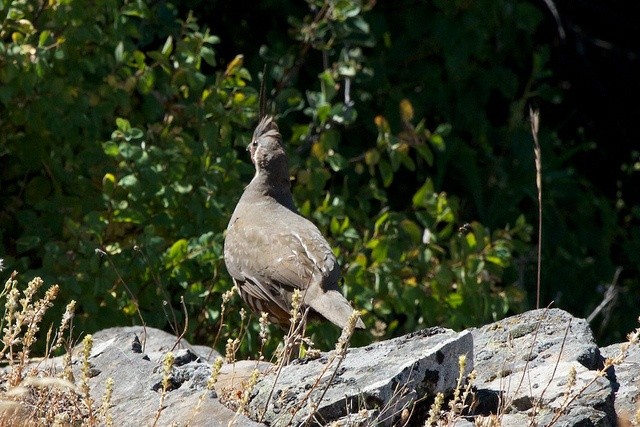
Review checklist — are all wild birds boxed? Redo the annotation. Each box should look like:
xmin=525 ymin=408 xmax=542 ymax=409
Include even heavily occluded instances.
xmin=222 ymin=113 xmax=367 ymax=366
